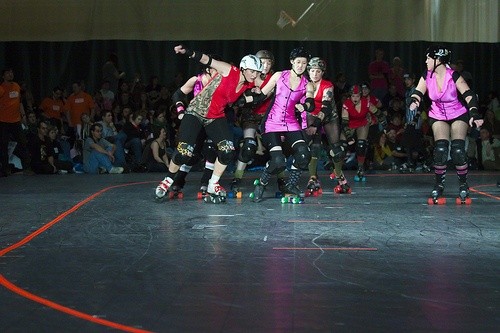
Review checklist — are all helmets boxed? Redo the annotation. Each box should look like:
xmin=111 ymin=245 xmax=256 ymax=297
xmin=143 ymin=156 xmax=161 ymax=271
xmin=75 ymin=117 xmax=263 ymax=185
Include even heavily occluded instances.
xmin=289 ymin=47 xmax=313 ymax=62
xmin=239 ymin=54 xmax=264 ymax=72
xmin=307 ymin=57 xmax=327 ymax=70
xmin=425 ymin=45 xmax=451 ymax=64
xmin=403 ymin=72 xmax=416 ymax=79
xmin=255 ymin=49 xmax=274 ymax=62
xmin=359 ymin=81 xmax=370 ymax=89
xmin=349 ymin=85 xmax=363 ymax=96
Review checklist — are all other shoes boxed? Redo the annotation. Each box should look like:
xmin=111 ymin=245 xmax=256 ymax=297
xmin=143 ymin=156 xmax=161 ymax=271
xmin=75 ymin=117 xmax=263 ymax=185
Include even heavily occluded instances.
xmin=385 ymin=165 xmax=430 ymax=172
xmin=0 ymin=164 xmax=155 ymax=176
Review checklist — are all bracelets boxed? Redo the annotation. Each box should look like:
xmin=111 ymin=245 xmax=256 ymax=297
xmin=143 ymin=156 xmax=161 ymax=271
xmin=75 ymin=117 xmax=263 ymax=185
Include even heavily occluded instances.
xmin=22 ymin=113 xmax=26 ymax=118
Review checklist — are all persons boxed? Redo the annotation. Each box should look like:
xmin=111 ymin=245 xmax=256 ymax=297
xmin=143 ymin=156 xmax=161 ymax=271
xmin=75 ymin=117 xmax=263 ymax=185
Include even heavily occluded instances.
xmin=409 ymin=47 xmax=484 ymax=198
xmin=101 ymin=52 xmax=127 ymax=105
xmin=226 ymin=48 xmax=500 ymax=174
xmin=156 ymin=44 xmax=262 ymax=198
xmin=304 ymin=56 xmax=348 ymax=191
xmin=329 ymin=84 xmax=388 ymax=181
xmin=230 ymin=50 xmax=305 ymax=197
xmin=172 ymin=54 xmax=228 ymax=195
xmin=7 ymin=72 xmax=208 ymax=176
xmin=232 ymin=47 xmax=315 ymax=200
xmin=0 ymin=65 xmax=35 ymax=177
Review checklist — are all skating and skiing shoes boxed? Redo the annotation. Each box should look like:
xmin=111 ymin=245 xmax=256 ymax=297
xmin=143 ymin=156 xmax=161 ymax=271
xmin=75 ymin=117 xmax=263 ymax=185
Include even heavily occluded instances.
xmin=305 ymin=176 xmax=322 ymax=197
xmin=334 ymin=176 xmax=351 ymax=194
xmin=153 ymin=177 xmax=174 ymax=202
xmin=330 ymin=169 xmax=337 ymax=180
xmin=428 ymin=186 xmax=446 ymax=205
xmin=197 ymin=179 xmax=209 ymax=199
xmin=169 ymin=178 xmax=185 ymax=199
xmin=455 ymin=183 xmax=472 ymax=205
xmin=354 ymin=168 xmax=366 ymax=182
xmin=204 ymin=180 xmax=226 ymax=204
xmin=228 ymin=179 xmax=242 ymax=199
xmin=280 ymin=183 xmax=304 ymax=204
xmin=248 ymin=178 xmax=268 ymax=202
